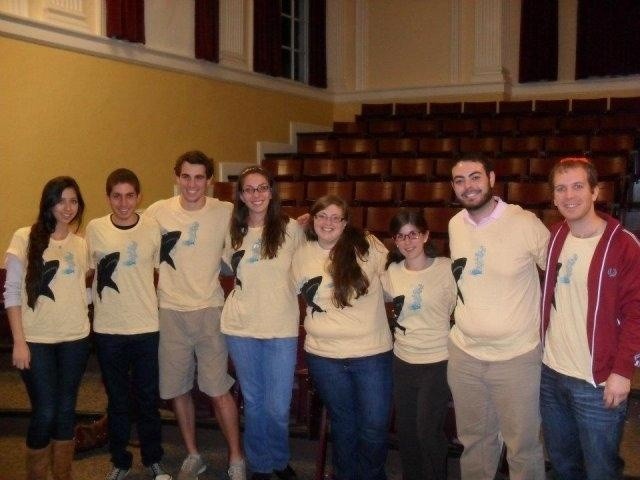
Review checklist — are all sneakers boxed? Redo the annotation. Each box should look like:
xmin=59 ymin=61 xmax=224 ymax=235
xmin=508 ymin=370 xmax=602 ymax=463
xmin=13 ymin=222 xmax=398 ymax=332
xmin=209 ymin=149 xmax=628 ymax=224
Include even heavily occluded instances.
xmin=227 ymin=459 xmax=247 ymax=480
xmin=250 ymin=471 xmax=271 ymax=479
xmin=104 ymin=466 xmax=132 ymax=480
xmin=149 ymin=461 xmax=173 ymax=480
xmin=176 ymin=455 xmax=208 ymax=480
xmin=272 ymin=464 xmax=297 ymax=480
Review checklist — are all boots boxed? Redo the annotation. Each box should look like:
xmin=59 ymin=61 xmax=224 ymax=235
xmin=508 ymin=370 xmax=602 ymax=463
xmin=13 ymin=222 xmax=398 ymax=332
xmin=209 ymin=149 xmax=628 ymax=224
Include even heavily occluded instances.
xmin=26 ymin=441 xmax=51 ymax=479
xmin=52 ymin=439 xmax=75 ymax=479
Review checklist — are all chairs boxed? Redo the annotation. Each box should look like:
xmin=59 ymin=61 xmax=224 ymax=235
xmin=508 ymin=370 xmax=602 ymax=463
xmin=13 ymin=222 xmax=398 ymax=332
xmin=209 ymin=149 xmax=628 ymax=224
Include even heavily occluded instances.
xmin=211 ymin=96 xmax=640 ymax=259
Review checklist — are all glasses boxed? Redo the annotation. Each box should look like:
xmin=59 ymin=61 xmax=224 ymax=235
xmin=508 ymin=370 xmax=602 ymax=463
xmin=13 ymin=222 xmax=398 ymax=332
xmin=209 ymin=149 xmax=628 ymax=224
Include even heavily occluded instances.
xmin=313 ymin=210 xmax=346 ymax=222
xmin=394 ymin=229 xmax=422 ymax=241
xmin=241 ymin=183 xmax=271 ymax=193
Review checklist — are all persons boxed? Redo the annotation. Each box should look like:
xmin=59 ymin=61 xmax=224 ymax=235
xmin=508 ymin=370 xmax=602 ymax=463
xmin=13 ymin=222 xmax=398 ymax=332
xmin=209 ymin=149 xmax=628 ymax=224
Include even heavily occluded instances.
xmin=141 ymin=150 xmax=313 ymax=480
xmin=540 ymin=158 xmax=640 ymax=480
xmin=84 ymin=168 xmax=169 ymax=480
xmin=379 ymin=210 xmax=456 ymax=480
xmin=3 ymin=175 xmax=90 ymax=480
xmin=445 ymin=151 xmax=549 ymax=480
xmin=218 ymin=166 xmax=309 ymax=480
xmin=288 ymin=195 xmax=395 ymax=480
